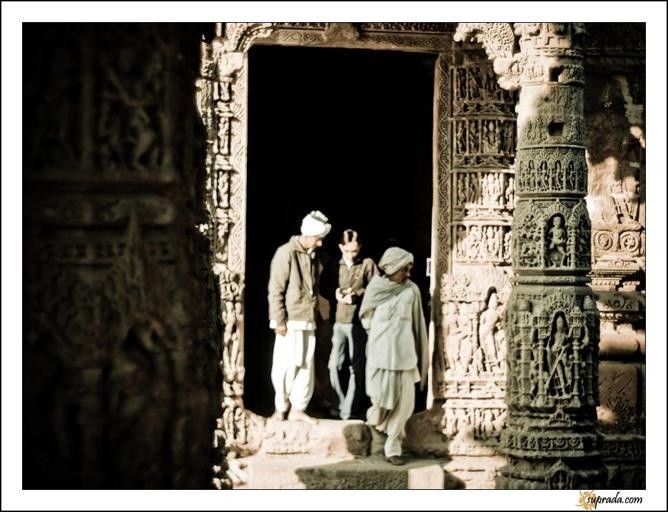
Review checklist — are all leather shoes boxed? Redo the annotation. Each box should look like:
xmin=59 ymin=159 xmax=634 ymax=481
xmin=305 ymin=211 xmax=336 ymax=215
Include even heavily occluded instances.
xmin=385 ymin=455 xmax=405 ymax=466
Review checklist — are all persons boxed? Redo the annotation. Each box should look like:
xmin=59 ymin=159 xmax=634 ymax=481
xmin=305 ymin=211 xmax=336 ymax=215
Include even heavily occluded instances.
xmin=546 ymin=316 xmax=575 ymax=397
xmin=357 ymin=244 xmax=429 ymax=467
xmin=437 ymin=52 xmax=515 ymax=443
xmin=547 ymin=213 xmax=567 ymax=268
xmin=314 ymin=223 xmax=380 ymax=418
xmin=266 ymin=210 xmax=333 ymax=426
